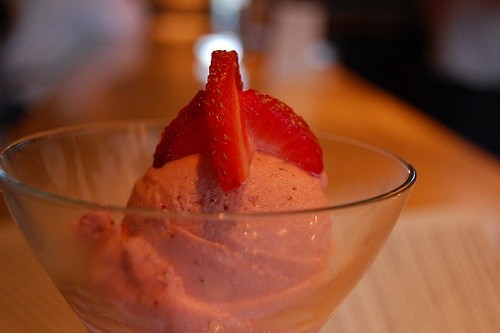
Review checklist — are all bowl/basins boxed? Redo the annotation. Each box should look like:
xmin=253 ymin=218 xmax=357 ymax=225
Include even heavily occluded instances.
xmin=0 ymin=119 xmax=416 ymax=332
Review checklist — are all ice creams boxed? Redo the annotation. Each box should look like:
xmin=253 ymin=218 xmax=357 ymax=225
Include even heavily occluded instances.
xmin=72 ymin=50 xmax=332 ymax=333
